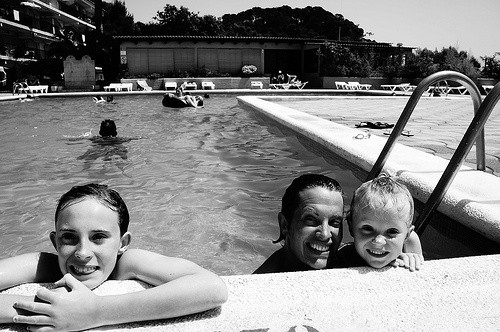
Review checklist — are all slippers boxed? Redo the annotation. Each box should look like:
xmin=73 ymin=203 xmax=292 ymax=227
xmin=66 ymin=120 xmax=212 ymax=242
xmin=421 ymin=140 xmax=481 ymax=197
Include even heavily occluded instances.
xmin=400 ymin=130 xmax=414 ymax=136
xmin=385 ymin=131 xmax=391 ymax=135
xmin=356 ymin=120 xmax=394 ymax=128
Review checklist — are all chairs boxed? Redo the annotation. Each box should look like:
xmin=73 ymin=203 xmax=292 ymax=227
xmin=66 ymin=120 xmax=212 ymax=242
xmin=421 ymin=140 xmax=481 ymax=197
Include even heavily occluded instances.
xmin=202 ymin=81 xmax=215 ymax=89
xmin=184 ymin=82 xmax=197 ymax=89
xmin=165 ymin=82 xmax=178 ymax=90
xmin=251 ymin=81 xmax=263 ymax=88
xmin=380 ymin=83 xmax=417 ymax=92
xmin=482 ymin=84 xmax=494 ymax=94
xmin=424 ymin=85 xmax=468 ymax=94
xmin=18 ymin=85 xmax=49 ymax=94
xmin=335 ymin=81 xmax=372 ymax=90
xmin=269 ymin=75 xmax=309 ymax=89
xmin=136 ymin=80 xmax=152 ymax=91
xmin=104 ymin=82 xmax=134 ymax=92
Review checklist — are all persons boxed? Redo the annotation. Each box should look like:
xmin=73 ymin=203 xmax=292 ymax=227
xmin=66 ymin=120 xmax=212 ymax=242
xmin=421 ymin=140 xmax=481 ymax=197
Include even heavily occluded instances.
xmin=93 ymin=95 xmax=114 ymax=104
xmin=169 ymin=86 xmax=203 ymax=108
xmin=0 ymin=183 xmax=228 ymax=332
xmin=99 ymin=119 xmax=118 ymax=137
xmin=251 ymin=172 xmax=424 ymax=275
